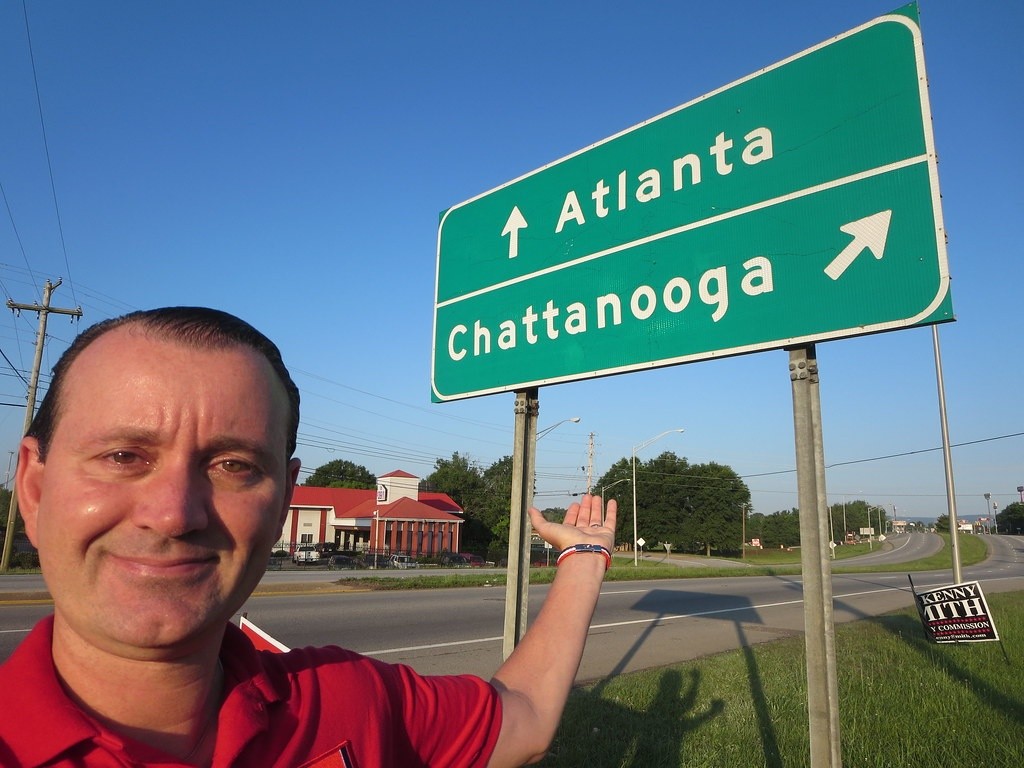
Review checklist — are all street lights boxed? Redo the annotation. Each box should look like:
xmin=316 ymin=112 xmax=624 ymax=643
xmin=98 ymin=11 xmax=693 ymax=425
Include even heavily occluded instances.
xmin=631 ymin=428 xmax=685 ymax=566
xmin=601 ymin=478 xmax=631 ymax=525
xmin=843 ymin=490 xmax=863 ymax=544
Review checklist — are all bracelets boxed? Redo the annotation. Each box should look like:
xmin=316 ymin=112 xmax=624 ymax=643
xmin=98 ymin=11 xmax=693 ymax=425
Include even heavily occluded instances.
xmin=556 ymin=543 xmax=611 ymax=570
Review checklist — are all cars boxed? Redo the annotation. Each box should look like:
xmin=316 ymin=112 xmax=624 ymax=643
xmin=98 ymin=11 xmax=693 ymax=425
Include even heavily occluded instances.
xmin=327 ymin=556 xmax=364 ymax=570
xmin=315 ymin=541 xmax=338 ymax=557
xmin=470 ymin=556 xmax=487 ymax=567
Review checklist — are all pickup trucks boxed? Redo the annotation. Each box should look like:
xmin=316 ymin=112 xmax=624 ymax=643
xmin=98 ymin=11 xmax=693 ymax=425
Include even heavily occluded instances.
xmin=390 ymin=554 xmax=417 ymax=569
xmin=292 ymin=545 xmax=321 ymax=566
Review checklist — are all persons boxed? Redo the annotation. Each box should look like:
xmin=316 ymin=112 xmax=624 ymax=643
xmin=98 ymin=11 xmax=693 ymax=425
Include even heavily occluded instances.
xmin=0 ymin=307 xmax=617 ymax=768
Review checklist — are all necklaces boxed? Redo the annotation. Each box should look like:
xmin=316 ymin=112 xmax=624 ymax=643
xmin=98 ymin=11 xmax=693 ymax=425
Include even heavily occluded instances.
xmin=182 ymin=657 xmax=224 ymax=761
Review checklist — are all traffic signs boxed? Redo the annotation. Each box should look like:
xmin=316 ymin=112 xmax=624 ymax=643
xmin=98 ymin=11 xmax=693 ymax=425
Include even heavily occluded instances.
xmin=429 ymin=1 xmax=958 ymax=408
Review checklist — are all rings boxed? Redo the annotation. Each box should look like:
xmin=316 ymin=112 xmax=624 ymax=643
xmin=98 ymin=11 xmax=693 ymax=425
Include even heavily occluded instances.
xmin=590 ymin=524 xmax=598 ymax=527
